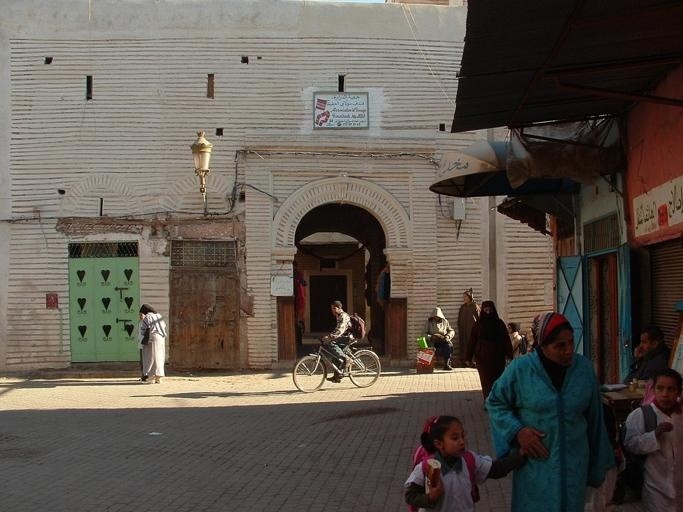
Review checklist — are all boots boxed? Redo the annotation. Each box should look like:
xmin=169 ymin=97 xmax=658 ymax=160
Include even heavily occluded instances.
xmin=443 ymin=359 xmax=452 ymax=370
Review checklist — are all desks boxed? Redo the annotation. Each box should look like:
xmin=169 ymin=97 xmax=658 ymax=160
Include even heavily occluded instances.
xmin=600 ymin=383 xmax=648 ymax=505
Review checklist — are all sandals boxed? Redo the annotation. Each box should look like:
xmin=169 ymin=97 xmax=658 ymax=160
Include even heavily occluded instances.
xmin=154 ymin=378 xmax=160 ymax=383
xmin=144 ymin=379 xmax=155 ymax=384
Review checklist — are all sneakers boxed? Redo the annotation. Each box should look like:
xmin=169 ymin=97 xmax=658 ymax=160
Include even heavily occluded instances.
xmin=140 ymin=376 xmax=148 ymax=381
xmin=342 ymin=358 xmax=353 ymax=368
xmin=327 ymin=376 xmax=341 ymax=383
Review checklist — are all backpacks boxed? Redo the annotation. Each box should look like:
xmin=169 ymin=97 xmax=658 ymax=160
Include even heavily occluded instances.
xmin=346 ymin=313 xmax=366 ymax=339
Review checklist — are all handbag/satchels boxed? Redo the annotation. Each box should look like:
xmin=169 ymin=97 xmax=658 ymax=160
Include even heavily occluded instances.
xmin=141 ymin=328 xmax=150 ymax=345
xmin=610 ymin=453 xmax=642 ymax=504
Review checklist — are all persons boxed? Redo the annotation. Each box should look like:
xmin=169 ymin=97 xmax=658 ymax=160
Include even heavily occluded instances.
xmin=506 ymin=320 xmax=528 ymax=360
xmin=621 ymin=326 xmax=671 ymax=384
xmin=619 ymin=367 xmax=682 ymax=512
xmin=464 ymin=299 xmax=514 ymax=412
xmin=294 ymin=317 xmax=305 ymax=358
xmin=137 ymin=311 xmax=148 ymax=382
xmin=137 ymin=303 xmax=168 ymax=385
xmin=320 ymin=300 xmax=357 ymax=383
xmin=422 ymin=306 xmax=455 ymax=370
xmin=456 ymin=287 xmax=481 ymax=367
xmin=401 ymin=413 xmax=529 ymax=511
xmin=484 ymin=311 xmax=616 ymax=512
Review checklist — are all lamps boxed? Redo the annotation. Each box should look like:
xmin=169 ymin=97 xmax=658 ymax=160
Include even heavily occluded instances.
xmin=187 ymin=129 xmax=215 ymax=213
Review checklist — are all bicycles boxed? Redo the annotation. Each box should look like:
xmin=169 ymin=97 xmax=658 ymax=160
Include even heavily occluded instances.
xmin=291 ymin=334 xmax=383 ymax=393
